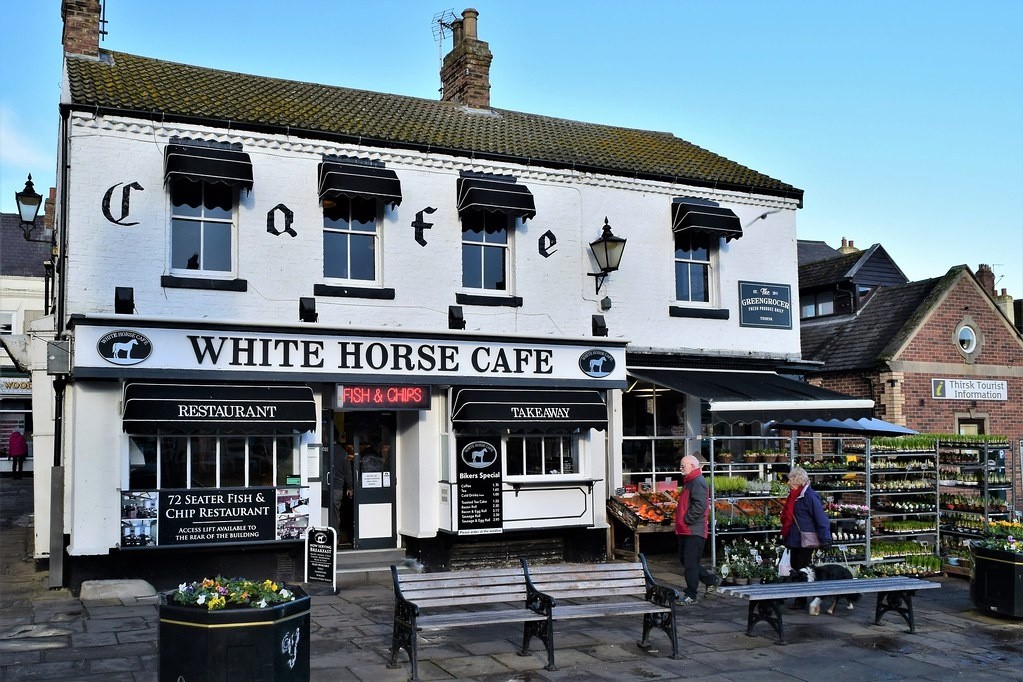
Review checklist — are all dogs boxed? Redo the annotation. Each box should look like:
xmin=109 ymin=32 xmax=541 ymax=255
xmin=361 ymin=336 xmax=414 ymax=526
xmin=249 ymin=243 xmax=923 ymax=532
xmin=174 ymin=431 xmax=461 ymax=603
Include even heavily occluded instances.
xmin=788 ymin=564 xmax=862 ymax=615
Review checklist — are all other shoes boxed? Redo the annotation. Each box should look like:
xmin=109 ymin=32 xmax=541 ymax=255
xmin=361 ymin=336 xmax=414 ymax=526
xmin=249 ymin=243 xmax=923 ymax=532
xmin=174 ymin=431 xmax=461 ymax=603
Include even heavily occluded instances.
xmin=13 ymin=475 xmax=22 ymax=480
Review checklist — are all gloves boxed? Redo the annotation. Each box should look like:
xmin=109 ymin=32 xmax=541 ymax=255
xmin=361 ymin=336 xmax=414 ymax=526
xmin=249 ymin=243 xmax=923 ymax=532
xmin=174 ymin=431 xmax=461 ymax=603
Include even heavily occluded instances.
xmin=820 ymin=542 xmax=832 ymax=552
xmin=8 ymin=455 xmax=11 ymax=460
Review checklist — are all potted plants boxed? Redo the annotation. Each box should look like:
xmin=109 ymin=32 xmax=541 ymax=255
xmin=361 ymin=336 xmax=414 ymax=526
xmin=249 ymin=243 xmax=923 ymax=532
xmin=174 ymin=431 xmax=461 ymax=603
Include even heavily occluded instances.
xmin=743 ymin=449 xmax=761 ymax=462
xmin=777 ymin=449 xmax=790 ymax=462
xmin=759 ymin=449 xmax=778 ymax=462
xmin=719 ymin=447 xmax=733 ymax=462
xmin=940 ymin=433 xmax=1010 ymax=568
xmin=705 ymin=472 xmax=790 ymax=529
xmin=795 ymin=436 xmax=937 ymax=576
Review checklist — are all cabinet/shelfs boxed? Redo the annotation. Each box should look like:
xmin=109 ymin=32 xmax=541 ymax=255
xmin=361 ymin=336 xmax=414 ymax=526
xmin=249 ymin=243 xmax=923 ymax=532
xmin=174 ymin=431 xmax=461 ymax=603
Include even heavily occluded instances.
xmin=791 ymin=435 xmax=869 ymax=567
xmin=867 ymin=440 xmax=939 ymax=575
xmin=684 ymin=435 xmax=791 ymax=586
xmin=940 ymin=435 xmax=1016 ymax=577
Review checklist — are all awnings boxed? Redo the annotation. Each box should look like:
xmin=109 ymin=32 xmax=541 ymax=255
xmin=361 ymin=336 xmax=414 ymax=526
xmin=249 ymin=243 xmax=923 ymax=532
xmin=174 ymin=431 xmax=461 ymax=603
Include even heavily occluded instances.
xmin=164 ymin=144 xmax=253 ymax=198
xmin=451 ymin=386 xmax=608 ymax=433
xmin=122 ymin=379 xmax=317 ymax=434
xmin=457 ymin=177 xmax=537 ymax=224
xmin=318 ymin=162 xmax=402 ymax=211
xmin=627 ymin=366 xmax=875 ymax=411
xmin=672 ymin=203 xmax=743 ymax=243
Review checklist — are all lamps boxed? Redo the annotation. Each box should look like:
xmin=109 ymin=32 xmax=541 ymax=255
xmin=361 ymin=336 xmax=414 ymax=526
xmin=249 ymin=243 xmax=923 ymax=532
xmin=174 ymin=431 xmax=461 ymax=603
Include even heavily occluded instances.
xmin=587 ymin=217 xmax=627 ymax=295
xmin=15 ymin=172 xmax=57 ymax=246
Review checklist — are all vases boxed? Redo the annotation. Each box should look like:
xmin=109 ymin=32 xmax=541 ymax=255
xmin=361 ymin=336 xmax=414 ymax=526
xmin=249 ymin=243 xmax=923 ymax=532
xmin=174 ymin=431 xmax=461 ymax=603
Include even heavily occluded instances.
xmin=726 ymin=577 xmax=733 ymax=582
xmin=750 ymin=578 xmax=763 ymax=583
xmin=735 ymin=578 xmax=748 ymax=584
xmin=156 ymin=580 xmax=313 ymax=682
xmin=967 ymin=539 xmax=1023 ymax=624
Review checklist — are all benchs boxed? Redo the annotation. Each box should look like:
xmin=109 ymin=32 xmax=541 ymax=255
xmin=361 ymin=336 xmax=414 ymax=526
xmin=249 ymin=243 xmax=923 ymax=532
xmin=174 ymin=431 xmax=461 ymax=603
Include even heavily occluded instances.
xmin=713 ymin=575 xmax=946 ymax=646
xmin=389 ymin=552 xmax=685 ymax=682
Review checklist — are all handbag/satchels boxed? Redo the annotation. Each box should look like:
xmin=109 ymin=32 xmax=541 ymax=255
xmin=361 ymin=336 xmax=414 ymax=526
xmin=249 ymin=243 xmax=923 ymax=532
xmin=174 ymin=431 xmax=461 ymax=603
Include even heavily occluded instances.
xmin=778 ymin=548 xmax=791 ymax=577
xmin=800 ymin=532 xmax=820 ymax=549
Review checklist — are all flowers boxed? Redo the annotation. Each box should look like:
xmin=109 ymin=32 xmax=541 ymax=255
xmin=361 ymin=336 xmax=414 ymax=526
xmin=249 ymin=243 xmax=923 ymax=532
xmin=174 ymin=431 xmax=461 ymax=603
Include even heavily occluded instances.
xmin=170 ymin=569 xmax=296 ymax=608
xmin=980 ymin=519 xmax=1023 ymax=553
xmin=719 ymin=535 xmax=783 ymax=577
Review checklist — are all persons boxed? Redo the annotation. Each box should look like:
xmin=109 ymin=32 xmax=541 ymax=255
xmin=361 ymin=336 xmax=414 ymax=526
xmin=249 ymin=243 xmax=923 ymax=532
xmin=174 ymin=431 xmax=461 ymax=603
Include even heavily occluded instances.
xmin=673 ymin=455 xmax=720 ymax=606
xmin=121 ymin=503 xmax=158 ymax=519
xmin=123 ymin=529 xmax=149 ymax=547
xmin=781 ymin=467 xmax=833 ymax=610
xmin=8 ymin=427 xmax=27 ymax=479
xmin=323 ymin=423 xmax=354 ymax=547
xmin=350 ymin=438 xmax=384 ymax=474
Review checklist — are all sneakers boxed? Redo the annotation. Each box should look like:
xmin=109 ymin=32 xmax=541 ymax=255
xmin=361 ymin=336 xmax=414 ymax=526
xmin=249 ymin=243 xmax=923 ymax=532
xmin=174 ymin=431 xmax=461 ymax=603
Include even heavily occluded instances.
xmin=676 ymin=596 xmax=698 ymax=606
xmin=704 ymin=581 xmax=719 ymax=598
